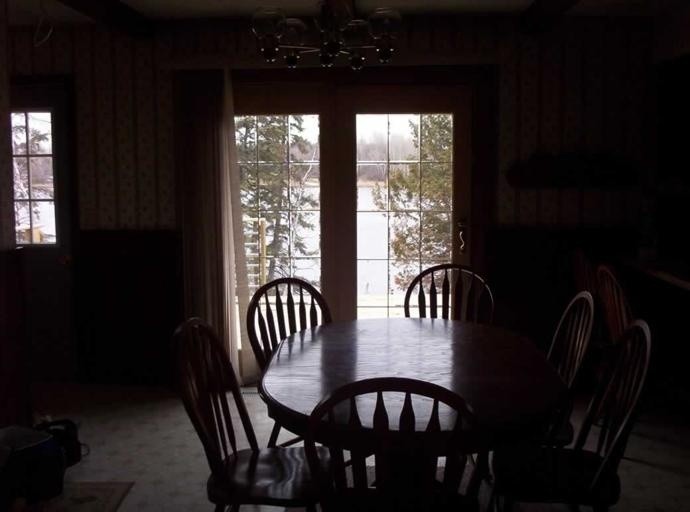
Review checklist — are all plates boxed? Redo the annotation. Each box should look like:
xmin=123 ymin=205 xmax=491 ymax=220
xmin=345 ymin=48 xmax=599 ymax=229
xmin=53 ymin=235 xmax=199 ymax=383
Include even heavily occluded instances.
xmin=0 ymin=424 xmax=53 ymax=452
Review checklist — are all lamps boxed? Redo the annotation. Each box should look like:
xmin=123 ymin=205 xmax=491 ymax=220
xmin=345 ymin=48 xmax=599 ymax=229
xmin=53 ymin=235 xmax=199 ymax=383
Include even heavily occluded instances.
xmin=251 ymin=0 xmax=403 ymax=70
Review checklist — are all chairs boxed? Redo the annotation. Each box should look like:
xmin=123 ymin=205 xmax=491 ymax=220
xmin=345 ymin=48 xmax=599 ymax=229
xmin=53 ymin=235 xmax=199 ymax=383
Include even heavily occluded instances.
xmin=171 ymin=264 xmax=653 ymax=512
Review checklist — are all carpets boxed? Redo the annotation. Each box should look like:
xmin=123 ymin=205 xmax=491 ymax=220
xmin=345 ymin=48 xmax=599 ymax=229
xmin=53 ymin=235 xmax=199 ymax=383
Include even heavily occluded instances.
xmin=31 ymin=481 xmax=135 ymax=512
